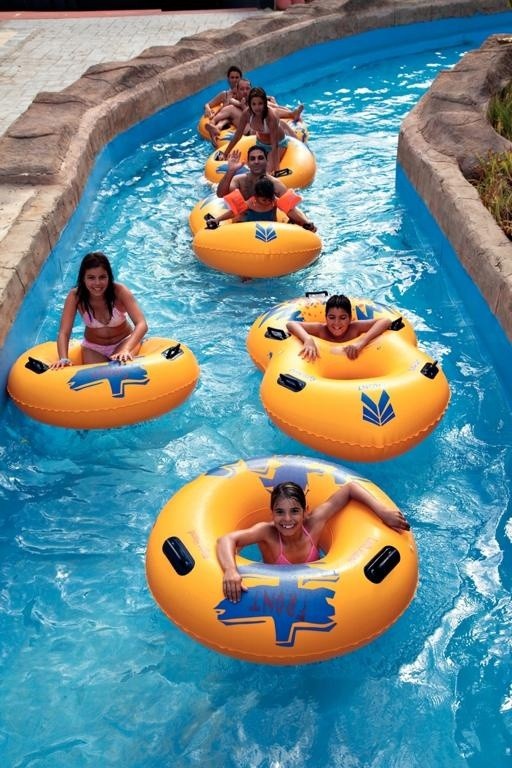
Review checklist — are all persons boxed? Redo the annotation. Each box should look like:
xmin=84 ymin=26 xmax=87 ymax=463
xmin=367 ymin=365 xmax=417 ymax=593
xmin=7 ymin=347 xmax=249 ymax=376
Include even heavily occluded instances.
xmin=215 ymin=479 xmax=411 ymax=604
xmin=48 ymin=252 xmax=147 ymax=371
xmin=203 ymin=66 xmax=318 ymax=286
xmin=286 ymin=295 xmax=393 ymax=363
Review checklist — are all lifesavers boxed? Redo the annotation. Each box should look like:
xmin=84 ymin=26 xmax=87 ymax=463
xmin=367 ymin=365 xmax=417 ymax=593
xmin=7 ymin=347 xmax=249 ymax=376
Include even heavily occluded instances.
xmin=249 ymin=292 xmax=451 ymax=464
xmin=146 ymin=454 xmax=417 ymax=664
xmin=8 ymin=337 xmax=198 ymax=428
xmin=188 ymin=98 xmax=320 ymax=278
